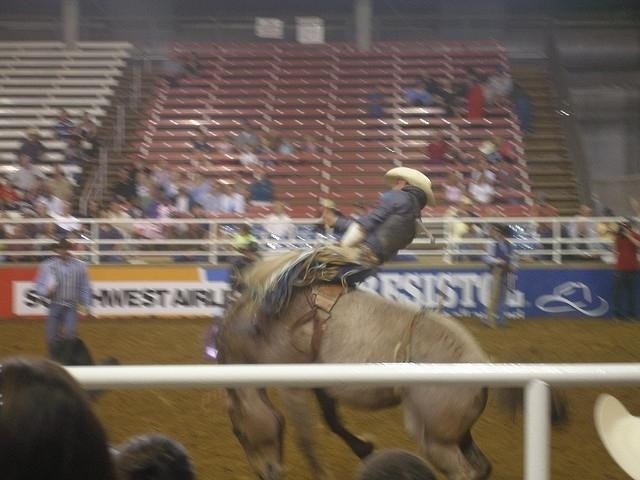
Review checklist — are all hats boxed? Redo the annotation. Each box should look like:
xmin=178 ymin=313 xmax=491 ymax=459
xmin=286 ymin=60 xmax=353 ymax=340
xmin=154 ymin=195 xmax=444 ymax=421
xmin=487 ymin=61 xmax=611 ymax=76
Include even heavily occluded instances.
xmin=594 ymin=392 xmax=639 ymax=479
xmin=384 ymin=167 xmax=437 ymax=207
xmin=52 ymin=238 xmax=73 ymax=249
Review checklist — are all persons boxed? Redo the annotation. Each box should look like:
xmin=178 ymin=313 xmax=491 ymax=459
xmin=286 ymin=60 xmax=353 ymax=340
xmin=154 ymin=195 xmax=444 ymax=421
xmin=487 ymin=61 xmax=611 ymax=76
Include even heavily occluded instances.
xmin=253 ymin=165 xmax=436 ymax=327
xmin=352 ymin=449 xmax=437 ymax=478
xmin=538 ymin=211 xmax=639 ymax=265
xmin=609 ymin=217 xmax=640 ymax=319
xmin=314 ymin=200 xmax=341 ymax=236
xmin=484 ymin=224 xmax=519 ymax=326
xmin=0 ymin=110 xmax=99 ymax=263
xmin=114 ymin=433 xmax=197 ymax=480
xmin=404 ymin=61 xmax=518 ymax=207
xmin=33 ymin=238 xmax=98 ymax=346
xmin=1 ymin=356 xmax=117 ymax=479
xmin=95 ymin=121 xmax=274 ymax=264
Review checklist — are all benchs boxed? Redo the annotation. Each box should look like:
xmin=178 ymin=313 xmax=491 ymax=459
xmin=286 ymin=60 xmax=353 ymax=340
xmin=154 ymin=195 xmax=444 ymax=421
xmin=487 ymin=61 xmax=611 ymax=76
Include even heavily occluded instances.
xmin=128 ymin=39 xmax=540 ymax=235
xmin=0 ymin=36 xmax=139 ymax=232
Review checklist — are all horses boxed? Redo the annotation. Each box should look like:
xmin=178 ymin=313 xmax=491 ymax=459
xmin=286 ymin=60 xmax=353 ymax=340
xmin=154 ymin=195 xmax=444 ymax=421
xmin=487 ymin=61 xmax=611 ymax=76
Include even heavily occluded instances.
xmin=217 ymin=250 xmax=572 ymax=479
